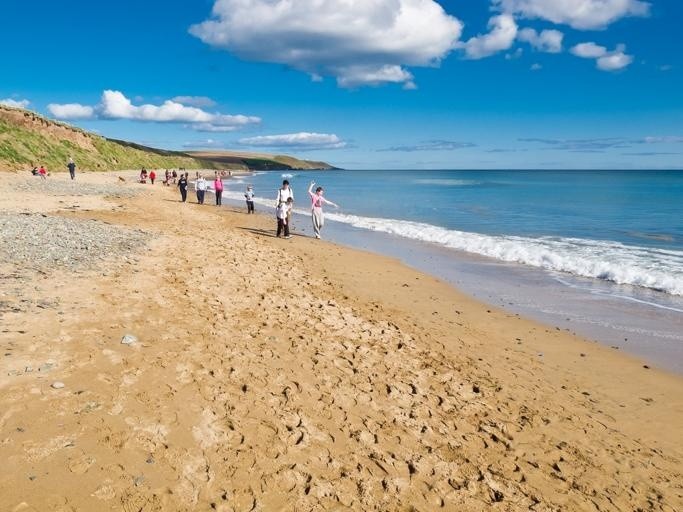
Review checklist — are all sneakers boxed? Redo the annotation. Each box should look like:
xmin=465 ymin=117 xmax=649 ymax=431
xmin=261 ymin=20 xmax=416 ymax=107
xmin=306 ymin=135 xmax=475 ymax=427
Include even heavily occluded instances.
xmin=277 ymin=233 xmax=292 ymax=239
xmin=316 ymin=235 xmax=321 ymax=239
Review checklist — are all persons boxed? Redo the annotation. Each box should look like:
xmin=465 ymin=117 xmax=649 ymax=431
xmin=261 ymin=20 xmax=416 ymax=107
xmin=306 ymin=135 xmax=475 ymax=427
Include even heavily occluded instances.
xmin=213 ymin=169 xmax=233 ymax=177
xmin=184 ymin=171 xmax=189 ymax=180
xmin=66 ymin=158 xmax=76 ymax=179
xmin=195 ymin=174 xmax=207 ymax=205
xmin=31 ymin=166 xmax=47 ymax=182
xmin=244 ymin=185 xmax=254 ymax=214
xmin=214 ymin=175 xmax=223 ymax=207
xmin=163 ymin=168 xmax=177 ymax=187
xmin=140 ymin=167 xmax=155 ymax=185
xmin=274 ymin=179 xmax=294 ymax=239
xmin=308 ymin=179 xmax=339 ymax=239
xmin=178 ymin=175 xmax=188 ymax=203
xmin=195 ymin=170 xmax=200 ymax=178
